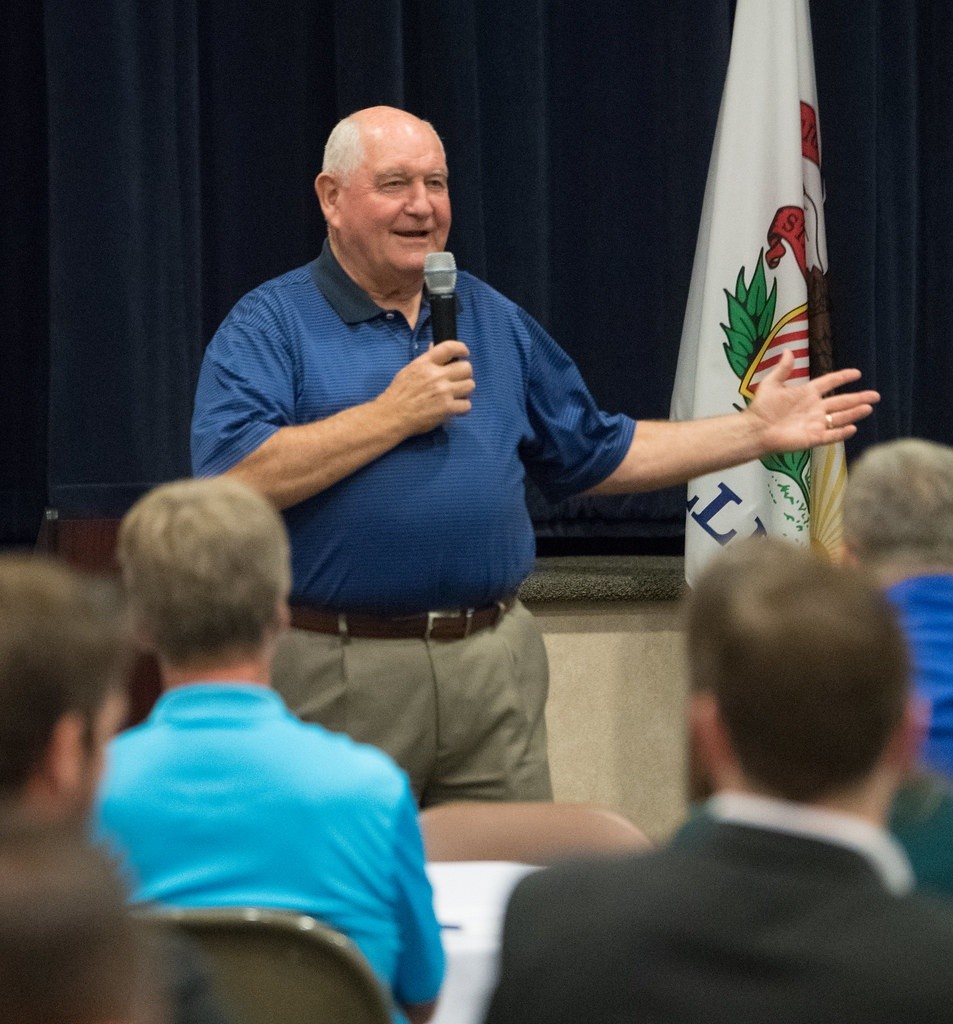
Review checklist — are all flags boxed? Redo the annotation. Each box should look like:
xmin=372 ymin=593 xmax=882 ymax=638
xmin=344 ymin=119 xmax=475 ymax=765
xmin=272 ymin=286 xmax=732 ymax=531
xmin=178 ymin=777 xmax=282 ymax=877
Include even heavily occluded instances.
xmin=668 ymin=1 xmax=829 ymax=588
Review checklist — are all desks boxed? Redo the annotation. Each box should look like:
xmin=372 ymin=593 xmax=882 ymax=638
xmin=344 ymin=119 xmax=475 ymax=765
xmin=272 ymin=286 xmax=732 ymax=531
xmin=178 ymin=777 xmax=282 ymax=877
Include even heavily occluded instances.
xmin=51 ymin=511 xmax=694 ymax=606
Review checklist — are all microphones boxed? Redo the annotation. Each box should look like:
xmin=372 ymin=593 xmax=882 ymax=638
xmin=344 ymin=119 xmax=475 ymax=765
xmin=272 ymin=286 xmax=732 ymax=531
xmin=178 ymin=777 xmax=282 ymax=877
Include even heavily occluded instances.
xmin=422 ymin=252 xmax=468 ymax=416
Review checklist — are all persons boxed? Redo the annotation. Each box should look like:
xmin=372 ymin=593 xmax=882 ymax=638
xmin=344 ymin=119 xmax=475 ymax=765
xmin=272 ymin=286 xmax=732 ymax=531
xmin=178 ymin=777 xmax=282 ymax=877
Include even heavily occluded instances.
xmin=189 ymin=103 xmax=882 ymax=811
xmin=92 ymin=472 xmax=448 ymax=1022
xmin=3 ymin=826 xmax=158 ymax=1020
xmin=840 ymin=437 xmax=952 ymax=781
xmin=3 ymin=554 xmax=134 ymax=834
xmin=479 ymin=532 xmax=952 ymax=1020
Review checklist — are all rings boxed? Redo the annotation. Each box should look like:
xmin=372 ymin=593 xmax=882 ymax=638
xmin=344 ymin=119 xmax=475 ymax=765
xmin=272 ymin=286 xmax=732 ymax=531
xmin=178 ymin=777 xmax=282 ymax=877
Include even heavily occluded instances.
xmin=825 ymin=415 xmax=833 ymax=429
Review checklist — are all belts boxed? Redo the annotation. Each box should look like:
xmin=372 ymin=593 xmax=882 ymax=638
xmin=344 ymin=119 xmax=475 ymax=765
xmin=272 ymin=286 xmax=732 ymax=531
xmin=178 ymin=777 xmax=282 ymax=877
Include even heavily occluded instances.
xmin=287 ymin=591 xmax=527 ymax=642
